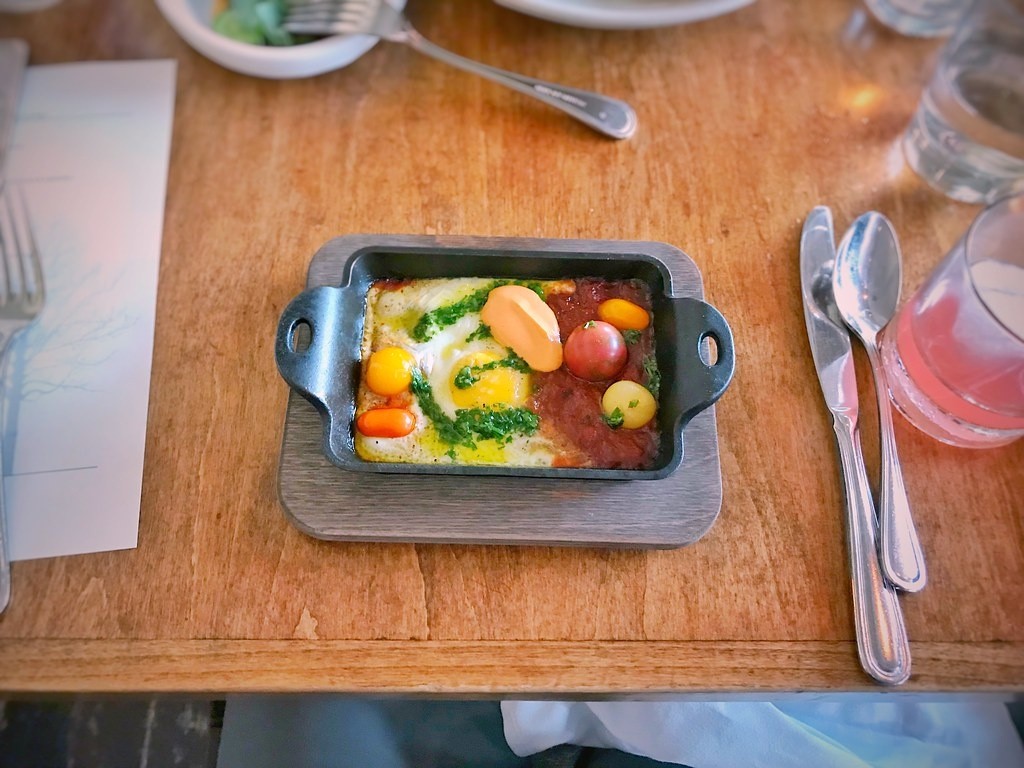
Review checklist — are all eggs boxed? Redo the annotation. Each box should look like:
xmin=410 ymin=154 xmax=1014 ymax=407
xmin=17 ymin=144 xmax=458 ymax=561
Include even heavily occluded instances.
xmin=356 ymin=277 xmax=564 ymax=467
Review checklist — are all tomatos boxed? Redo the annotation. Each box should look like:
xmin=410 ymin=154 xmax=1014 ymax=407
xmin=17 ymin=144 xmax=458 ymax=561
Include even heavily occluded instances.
xmin=356 ymin=299 xmax=655 ymax=438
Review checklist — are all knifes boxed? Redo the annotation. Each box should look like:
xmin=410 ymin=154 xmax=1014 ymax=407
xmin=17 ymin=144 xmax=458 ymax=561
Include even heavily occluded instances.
xmin=799 ymin=206 xmax=913 ymax=686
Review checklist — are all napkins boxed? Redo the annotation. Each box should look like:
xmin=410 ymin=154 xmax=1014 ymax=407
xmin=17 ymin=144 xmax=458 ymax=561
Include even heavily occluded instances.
xmin=0 ymin=59 xmax=175 ymax=563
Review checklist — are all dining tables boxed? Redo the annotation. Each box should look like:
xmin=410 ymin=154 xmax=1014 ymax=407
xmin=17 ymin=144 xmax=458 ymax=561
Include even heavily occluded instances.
xmin=0 ymin=0 xmax=1024 ymax=697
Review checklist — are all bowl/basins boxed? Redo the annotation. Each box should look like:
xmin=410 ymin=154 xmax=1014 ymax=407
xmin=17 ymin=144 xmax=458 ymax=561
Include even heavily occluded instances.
xmin=155 ymin=0 xmax=405 ymax=79
xmin=496 ymin=0 xmax=756 ymax=28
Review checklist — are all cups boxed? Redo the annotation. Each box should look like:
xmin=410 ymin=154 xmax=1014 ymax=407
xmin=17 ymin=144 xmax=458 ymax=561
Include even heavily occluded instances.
xmin=900 ymin=0 xmax=1024 ymax=202
xmin=863 ymin=0 xmax=966 ymax=37
xmin=882 ymin=191 xmax=1024 ymax=450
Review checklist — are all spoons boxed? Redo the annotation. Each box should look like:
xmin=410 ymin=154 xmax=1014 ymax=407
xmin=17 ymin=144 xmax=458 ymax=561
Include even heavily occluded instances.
xmin=832 ymin=209 xmax=927 ymax=594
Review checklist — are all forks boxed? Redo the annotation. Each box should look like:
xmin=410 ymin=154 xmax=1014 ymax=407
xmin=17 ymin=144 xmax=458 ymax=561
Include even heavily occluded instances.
xmin=283 ymin=0 xmax=635 ymax=139
xmin=0 ymin=181 xmax=46 ymax=616
